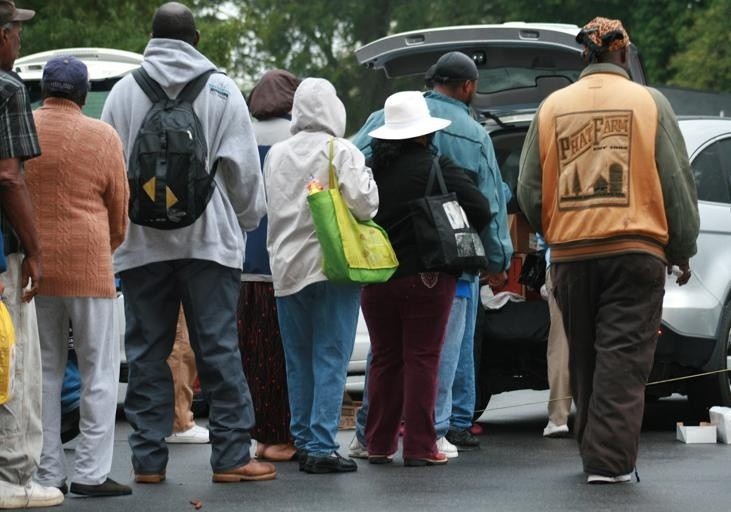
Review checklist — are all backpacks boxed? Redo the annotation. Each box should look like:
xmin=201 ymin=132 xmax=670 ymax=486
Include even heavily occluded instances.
xmin=127 ymin=67 xmax=219 ymax=230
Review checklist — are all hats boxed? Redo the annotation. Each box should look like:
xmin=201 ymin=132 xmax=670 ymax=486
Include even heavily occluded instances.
xmin=42 ymin=56 xmax=88 ymax=99
xmin=1 ymin=2 xmax=36 ymax=25
xmin=368 ymin=91 xmax=451 ymax=140
xmin=247 ymin=68 xmax=300 ymax=119
xmin=434 ymin=51 xmax=478 ymax=82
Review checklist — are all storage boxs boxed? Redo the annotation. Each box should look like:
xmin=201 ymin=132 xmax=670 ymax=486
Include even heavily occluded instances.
xmin=507 ymin=210 xmax=535 ymax=253
xmin=491 ymin=252 xmax=536 ymax=302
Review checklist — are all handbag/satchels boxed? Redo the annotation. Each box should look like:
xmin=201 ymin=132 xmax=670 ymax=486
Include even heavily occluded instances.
xmin=408 ymin=156 xmax=488 ymax=273
xmin=518 ymin=249 xmax=547 ymax=291
xmin=60 ymin=343 xmax=81 ymax=443
xmin=307 ymin=139 xmax=400 ymax=286
xmin=2 ymin=296 xmax=16 ymax=404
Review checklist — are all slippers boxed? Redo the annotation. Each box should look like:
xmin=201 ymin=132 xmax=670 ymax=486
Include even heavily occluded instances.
xmin=254 ymin=449 xmax=304 ymax=462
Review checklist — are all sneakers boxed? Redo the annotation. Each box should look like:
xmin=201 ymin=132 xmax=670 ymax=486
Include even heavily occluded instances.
xmin=446 ymin=429 xmax=480 ymax=450
xmin=0 ymin=482 xmax=65 ymax=508
xmin=164 ymin=426 xmax=210 ymax=443
xmin=435 ymin=437 xmax=458 ymax=458
xmin=348 ymin=435 xmax=369 ymax=458
xmin=587 ymin=472 xmax=631 ymax=483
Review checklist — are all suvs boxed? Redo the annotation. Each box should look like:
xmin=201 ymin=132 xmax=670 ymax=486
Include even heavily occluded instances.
xmin=353 ymin=19 xmax=731 ymax=434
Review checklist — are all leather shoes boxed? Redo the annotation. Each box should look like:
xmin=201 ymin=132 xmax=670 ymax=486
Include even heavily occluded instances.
xmin=403 ymin=450 xmax=447 ymax=466
xmin=213 ymin=451 xmax=277 ymax=482
xmin=368 ymin=454 xmax=393 ymax=464
xmin=135 ymin=470 xmax=166 ymax=483
xmin=542 ymin=420 xmax=569 ymax=436
xmin=302 ymin=450 xmax=357 ymax=474
xmin=71 ymin=478 xmax=131 ymax=497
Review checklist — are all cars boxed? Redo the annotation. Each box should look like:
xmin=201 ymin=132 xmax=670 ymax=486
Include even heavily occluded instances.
xmin=9 ymin=45 xmax=372 ymax=413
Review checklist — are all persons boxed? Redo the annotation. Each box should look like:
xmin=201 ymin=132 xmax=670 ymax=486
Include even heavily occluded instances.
xmin=542 ymin=248 xmax=574 ymax=439
xmin=422 ymin=62 xmax=512 ymax=449
xmin=100 ymin=0 xmax=276 ymax=483
xmin=236 ymin=67 xmax=301 ymax=464
xmin=0 ymin=0 xmax=64 ymax=509
xmin=515 ymin=14 xmax=701 ymax=485
xmin=359 ymin=89 xmax=492 ymax=466
xmin=18 ymin=53 xmax=132 ymax=498
xmin=262 ymin=76 xmax=380 ymax=475
xmin=348 ymin=51 xmax=514 ymax=461
